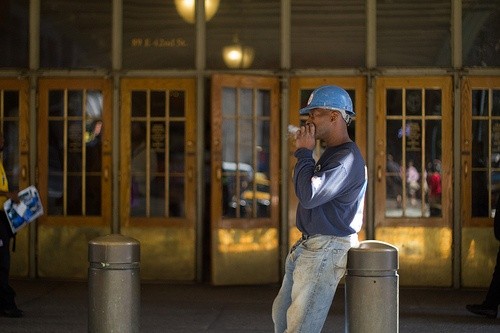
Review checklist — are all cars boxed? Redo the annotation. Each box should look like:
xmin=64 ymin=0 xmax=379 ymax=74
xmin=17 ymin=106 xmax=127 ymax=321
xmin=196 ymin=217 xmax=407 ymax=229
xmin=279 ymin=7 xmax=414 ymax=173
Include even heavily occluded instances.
xmin=77 ymin=128 xmax=254 ymax=212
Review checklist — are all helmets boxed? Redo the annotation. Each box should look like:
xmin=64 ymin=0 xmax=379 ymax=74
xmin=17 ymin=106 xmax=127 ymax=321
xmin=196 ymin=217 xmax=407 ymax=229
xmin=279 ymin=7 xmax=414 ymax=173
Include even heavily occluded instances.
xmin=299 ymin=85 xmax=355 ymax=119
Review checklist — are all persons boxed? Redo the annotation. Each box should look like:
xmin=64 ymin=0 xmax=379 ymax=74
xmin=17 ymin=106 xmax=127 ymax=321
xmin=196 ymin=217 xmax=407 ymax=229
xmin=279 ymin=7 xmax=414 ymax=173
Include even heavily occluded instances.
xmin=0 ymin=133 xmax=24 ymax=318
xmin=384 ymin=154 xmax=442 ymax=217
xmin=273 ymin=85 xmax=368 ymax=333
xmin=465 ymin=194 xmax=500 ymax=317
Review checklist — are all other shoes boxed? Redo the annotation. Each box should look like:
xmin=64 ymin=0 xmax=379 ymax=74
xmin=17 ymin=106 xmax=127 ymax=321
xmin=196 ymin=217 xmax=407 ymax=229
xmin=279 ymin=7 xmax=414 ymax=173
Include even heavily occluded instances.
xmin=0 ymin=306 xmax=24 ymax=317
xmin=465 ymin=303 xmax=498 ymax=319
